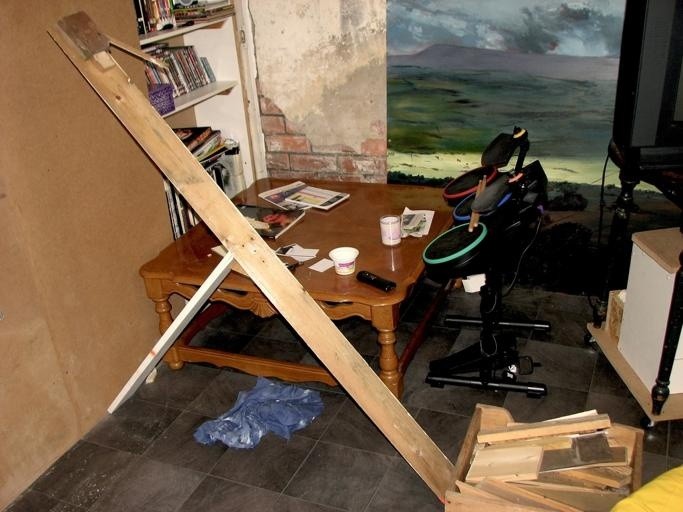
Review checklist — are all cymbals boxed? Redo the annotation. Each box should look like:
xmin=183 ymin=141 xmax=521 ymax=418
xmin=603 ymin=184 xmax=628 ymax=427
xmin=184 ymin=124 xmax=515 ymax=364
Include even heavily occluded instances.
xmin=471 ymin=175 xmax=509 ymax=213
xmin=481 ymin=133 xmax=518 ymax=167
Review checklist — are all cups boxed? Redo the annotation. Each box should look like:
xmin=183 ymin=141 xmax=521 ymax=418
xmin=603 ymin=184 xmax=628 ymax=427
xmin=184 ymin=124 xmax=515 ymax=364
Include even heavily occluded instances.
xmin=328 ymin=247 xmax=359 ymax=275
xmin=379 ymin=214 xmax=401 ymax=246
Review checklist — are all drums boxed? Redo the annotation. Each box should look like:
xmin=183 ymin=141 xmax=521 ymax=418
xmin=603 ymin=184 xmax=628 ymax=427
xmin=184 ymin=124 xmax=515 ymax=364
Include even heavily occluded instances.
xmin=423 ymin=223 xmax=488 ymax=264
xmin=453 ymin=189 xmax=512 ymax=221
xmin=443 ymin=166 xmax=497 ymax=198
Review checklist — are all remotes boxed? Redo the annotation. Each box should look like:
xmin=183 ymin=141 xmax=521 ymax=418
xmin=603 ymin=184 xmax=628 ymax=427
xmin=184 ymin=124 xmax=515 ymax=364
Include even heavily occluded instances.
xmin=357 ymin=271 xmax=396 ymax=293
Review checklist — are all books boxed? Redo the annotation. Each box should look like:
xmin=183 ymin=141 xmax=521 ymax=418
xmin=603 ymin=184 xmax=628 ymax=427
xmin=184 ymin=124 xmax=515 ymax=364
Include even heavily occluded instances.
xmin=234 ymin=203 xmax=306 ymax=242
xmin=132 ymin=1 xmax=248 ymax=243
xmin=287 ymin=186 xmax=350 ymax=212
xmin=257 ymin=179 xmax=316 ymax=212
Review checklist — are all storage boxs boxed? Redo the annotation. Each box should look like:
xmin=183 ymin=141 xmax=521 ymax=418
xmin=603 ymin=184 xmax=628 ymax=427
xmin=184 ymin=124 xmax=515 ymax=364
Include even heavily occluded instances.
xmin=618 ymin=225 xmax=681 ymax=396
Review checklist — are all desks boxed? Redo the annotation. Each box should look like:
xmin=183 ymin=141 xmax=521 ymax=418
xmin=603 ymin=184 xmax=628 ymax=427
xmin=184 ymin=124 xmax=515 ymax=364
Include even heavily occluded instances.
xmin=139 ymin=179 xmax=456 ymax=401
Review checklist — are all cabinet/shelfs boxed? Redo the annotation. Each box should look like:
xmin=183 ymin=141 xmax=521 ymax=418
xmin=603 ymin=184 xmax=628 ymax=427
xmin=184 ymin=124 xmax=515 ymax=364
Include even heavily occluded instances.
xmin=132 ymin=1 xmax=258 ymax=241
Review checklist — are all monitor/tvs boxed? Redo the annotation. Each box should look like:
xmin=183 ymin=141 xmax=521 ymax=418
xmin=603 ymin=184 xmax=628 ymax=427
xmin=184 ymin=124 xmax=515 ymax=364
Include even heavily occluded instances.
xmin=611 ymin=0 xmax=683 ymax=170
